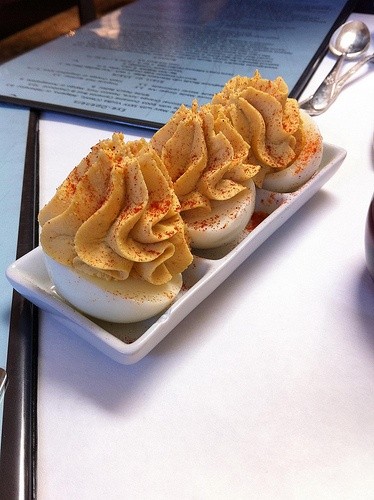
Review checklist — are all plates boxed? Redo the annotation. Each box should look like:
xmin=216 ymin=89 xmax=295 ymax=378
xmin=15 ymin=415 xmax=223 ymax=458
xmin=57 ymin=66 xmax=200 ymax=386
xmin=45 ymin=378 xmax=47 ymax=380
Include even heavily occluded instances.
xmin=5 ymin=137 xmax=349 ymax=368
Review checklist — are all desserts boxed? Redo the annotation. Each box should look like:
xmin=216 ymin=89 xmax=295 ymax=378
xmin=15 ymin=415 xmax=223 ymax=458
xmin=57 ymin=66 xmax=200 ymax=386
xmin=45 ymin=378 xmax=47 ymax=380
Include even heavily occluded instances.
xmin=35 ymin=67 xmax=324 ymax=325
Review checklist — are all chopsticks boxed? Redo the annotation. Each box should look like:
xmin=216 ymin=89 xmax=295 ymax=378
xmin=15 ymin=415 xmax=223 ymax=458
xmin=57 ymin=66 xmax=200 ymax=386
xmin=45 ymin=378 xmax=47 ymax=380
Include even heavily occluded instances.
xmin=0 ymin=106 xmax=43 ymax=500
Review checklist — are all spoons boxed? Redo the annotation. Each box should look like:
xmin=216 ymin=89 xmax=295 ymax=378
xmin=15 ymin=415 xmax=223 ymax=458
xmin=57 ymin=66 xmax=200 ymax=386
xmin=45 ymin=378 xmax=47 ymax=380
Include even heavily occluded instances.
xmin=312 ymin=18 xmax=371 ymax=109
